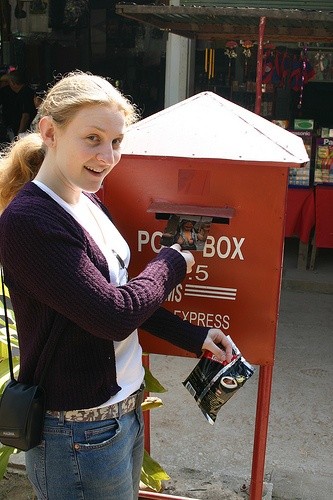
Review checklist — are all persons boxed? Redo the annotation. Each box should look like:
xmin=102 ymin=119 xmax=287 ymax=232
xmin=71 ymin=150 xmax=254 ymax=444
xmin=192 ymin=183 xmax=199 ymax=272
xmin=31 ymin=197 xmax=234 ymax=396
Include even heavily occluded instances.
xmin=0 ymin=73 xmax=234 ymax=500
xmin=0 ymin=32 xmax=32 ymax=143
xmin=162 ymin=220 xmax=208 ymax=250
xmin=5 ymin=70 xmax=38 ymax=142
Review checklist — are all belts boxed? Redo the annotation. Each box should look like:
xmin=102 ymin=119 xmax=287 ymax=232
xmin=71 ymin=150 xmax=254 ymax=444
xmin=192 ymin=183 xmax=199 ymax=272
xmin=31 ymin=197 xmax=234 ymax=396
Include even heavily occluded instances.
xmin=49 ymin=387 xmax=141 ymax=423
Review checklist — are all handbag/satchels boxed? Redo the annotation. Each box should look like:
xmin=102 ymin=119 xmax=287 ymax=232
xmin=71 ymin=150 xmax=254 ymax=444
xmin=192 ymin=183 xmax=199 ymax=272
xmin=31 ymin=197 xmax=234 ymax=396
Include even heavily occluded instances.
xmin=0 ymin=380 xmax=46 ymax=451
